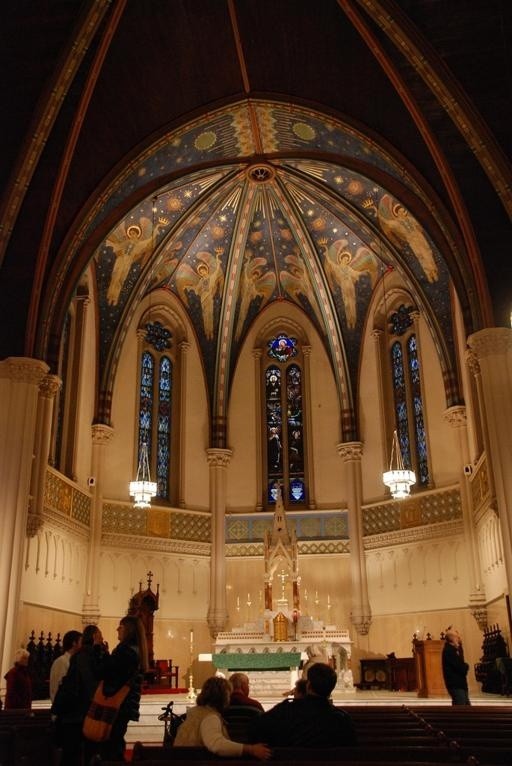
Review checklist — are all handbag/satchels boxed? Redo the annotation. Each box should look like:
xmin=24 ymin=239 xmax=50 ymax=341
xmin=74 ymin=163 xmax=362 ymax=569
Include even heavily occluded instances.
xmin=82 ymin=679 xmax=130 ymax=743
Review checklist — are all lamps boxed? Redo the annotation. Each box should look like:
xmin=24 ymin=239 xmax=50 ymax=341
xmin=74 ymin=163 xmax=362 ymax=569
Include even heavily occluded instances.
xmin=129 ymin=195 xmax=157 ymax=509
xmin=373 ymin=187 xmax=416 ymax=500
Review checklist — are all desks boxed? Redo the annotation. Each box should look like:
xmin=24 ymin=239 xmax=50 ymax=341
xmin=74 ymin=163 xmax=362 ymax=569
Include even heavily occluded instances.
xmin=211 ymin=652 xmax=301 ymax=700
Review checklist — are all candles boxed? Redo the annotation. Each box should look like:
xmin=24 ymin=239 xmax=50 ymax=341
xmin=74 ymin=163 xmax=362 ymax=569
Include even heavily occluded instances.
xmin=237 ymin=595 xmax=239 ymax=606
xmin=328 ymin=594 xmax=330 ymax=605
xmin=305 ymin=588 xmax=306 ymax=596
xmin=323 ymin=630 xmax=325 ymax=640
xmin=248 ymin=592 xmax=250 ymax=601
xmin=316 ymin=591 xmax=317 ymax=600
xmin=260 ymin=591 xmax=261 ymax=600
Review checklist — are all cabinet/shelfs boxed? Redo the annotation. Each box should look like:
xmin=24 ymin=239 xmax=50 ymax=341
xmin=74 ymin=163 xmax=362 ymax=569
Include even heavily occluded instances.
xmin=359 ymin=657 xmax=419 ymax=691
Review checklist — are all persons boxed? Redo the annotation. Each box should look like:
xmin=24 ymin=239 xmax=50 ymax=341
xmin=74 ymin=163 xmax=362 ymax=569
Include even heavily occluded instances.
xmin=228 ymin=672 xmax=267 ymax=714
xmin=441 ymin=629 xmax=473 ymax=706
xmin=51 ymin=624 xmax=110 ymax=765
xmin=365 ymin=204 xmax=441 ymax=285
xmin=3 ymin=647 xmax=35 ymax=711
xmin=232 ymin=253 xmax=266 ymax=343
xmin=101 ymin=614 xmax=150 ymax=766
xmin=106 ymin=218 xmax=172 ymax=307
xmin=247 ymin=662 xmax=356 ymax=749
xmin=181 ymin=249 xmax=227 ymax=342
xmin=292 ymin=249 xmax=328 ymax=338
xmin=171 ymin=674 xmax=272 ymax=763
xmin=47 ymin=628 xmax=82 ymax=706
xmin=292 ymin=678 xmax=306 ymax=699
xmin=322 ymin=249 xmax=371 ymax=332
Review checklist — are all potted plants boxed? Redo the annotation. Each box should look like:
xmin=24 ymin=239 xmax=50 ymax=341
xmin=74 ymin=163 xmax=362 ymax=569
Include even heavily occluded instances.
xmin=191 ymin=632 xmax=193 ymax=643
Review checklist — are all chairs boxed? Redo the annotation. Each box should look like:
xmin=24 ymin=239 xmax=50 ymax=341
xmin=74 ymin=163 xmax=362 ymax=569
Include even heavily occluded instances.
xmin=145 ymin=659 xmax=179 ymax=688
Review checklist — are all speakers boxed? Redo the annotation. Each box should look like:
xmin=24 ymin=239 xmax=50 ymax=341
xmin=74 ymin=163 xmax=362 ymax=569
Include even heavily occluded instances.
xmin=464 ymin=465 xmax=472 ymax=475
xmin=88 ymin=477 xmax=95 ymax=486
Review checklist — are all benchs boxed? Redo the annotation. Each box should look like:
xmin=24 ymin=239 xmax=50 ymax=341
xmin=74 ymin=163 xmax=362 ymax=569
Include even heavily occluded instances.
xmin=0 ymin=708 xmax=106 ymax=766
xmin=129 ymin=706 xmax=512 ymax=766
